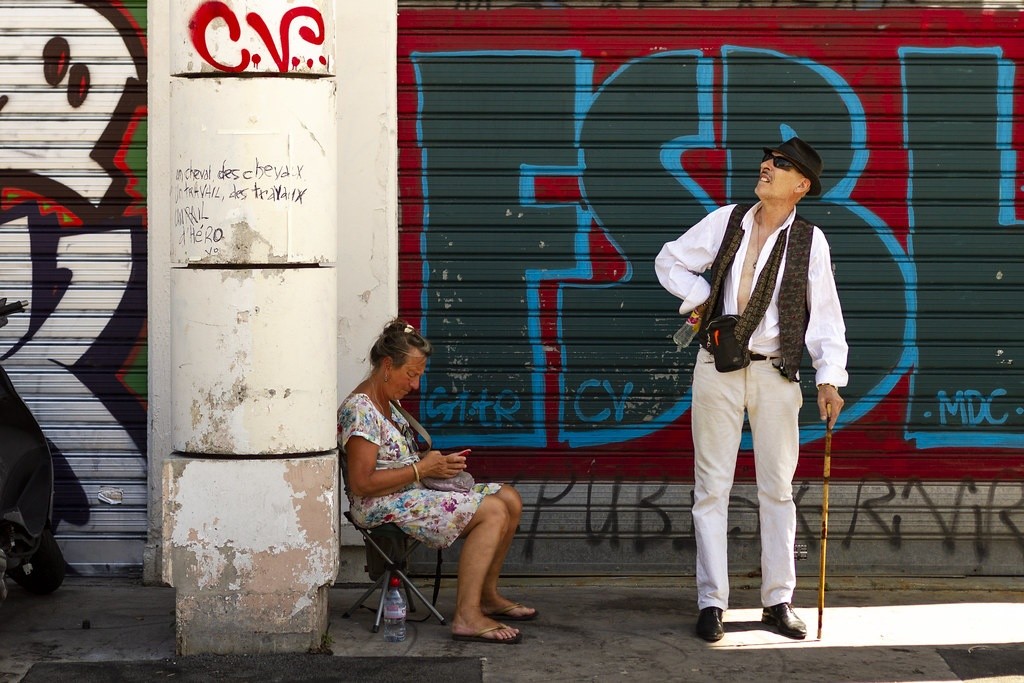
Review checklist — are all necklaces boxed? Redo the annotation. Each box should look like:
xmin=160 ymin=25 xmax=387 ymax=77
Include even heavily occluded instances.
xmin=753 ymin=209 xmax=761 ymax=270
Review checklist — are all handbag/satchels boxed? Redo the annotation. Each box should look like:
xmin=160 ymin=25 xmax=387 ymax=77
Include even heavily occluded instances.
xmin=421 ymin=469 xmax=475 ymax=493
xmin=705 ymin=313 xmax=751 ymax=372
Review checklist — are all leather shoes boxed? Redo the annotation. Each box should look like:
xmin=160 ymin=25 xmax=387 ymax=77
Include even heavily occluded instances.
xmin=696 ymin=606 xmax=725 ymax=640
xmin=762 ymin=602 xmax=806 ymax=637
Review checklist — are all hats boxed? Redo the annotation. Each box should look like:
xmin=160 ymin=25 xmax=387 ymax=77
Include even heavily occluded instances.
xmin=763 ymin=136 xmax=825 ymax=197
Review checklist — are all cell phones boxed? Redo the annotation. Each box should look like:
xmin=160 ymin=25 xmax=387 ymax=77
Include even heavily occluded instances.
xmin=448 ymin=449 xmax=472 ymax=458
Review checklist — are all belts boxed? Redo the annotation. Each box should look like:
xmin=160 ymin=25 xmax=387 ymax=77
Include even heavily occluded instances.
xmin=700 ymin=343 xmax=779 ymax=361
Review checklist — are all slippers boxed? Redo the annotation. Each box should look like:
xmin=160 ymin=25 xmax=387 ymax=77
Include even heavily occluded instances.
xmin=453 ymin=622 xmax=523 ymax=643
xmin=484 ymin=601 xmax=540 ymax=620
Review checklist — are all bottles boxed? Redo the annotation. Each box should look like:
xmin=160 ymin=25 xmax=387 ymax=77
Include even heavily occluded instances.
xmin=673 ymin=309 xmax=703 ymax=349
xmin=383 ymin=578 xmax=408 ymax=641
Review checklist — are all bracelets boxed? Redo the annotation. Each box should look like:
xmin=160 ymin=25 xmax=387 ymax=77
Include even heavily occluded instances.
xmin=818 ymin=383 xmax=838 ymax=391
xmin=412 ymin=464 xmax=420 ymax=483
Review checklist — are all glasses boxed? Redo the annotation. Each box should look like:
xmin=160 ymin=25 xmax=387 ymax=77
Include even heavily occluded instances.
xmin=761 ymin=152 xmax=800 ymax=173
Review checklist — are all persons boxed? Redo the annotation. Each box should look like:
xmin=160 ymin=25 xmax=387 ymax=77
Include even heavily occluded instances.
xmin=654 ymin=140 xmax=849 ymax=642
xmin=337 ymin=316 xmax=540 ymax=645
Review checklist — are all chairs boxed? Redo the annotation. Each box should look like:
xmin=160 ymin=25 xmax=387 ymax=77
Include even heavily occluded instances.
xmin=334 ymin=458 xmax=448 ymax=634
